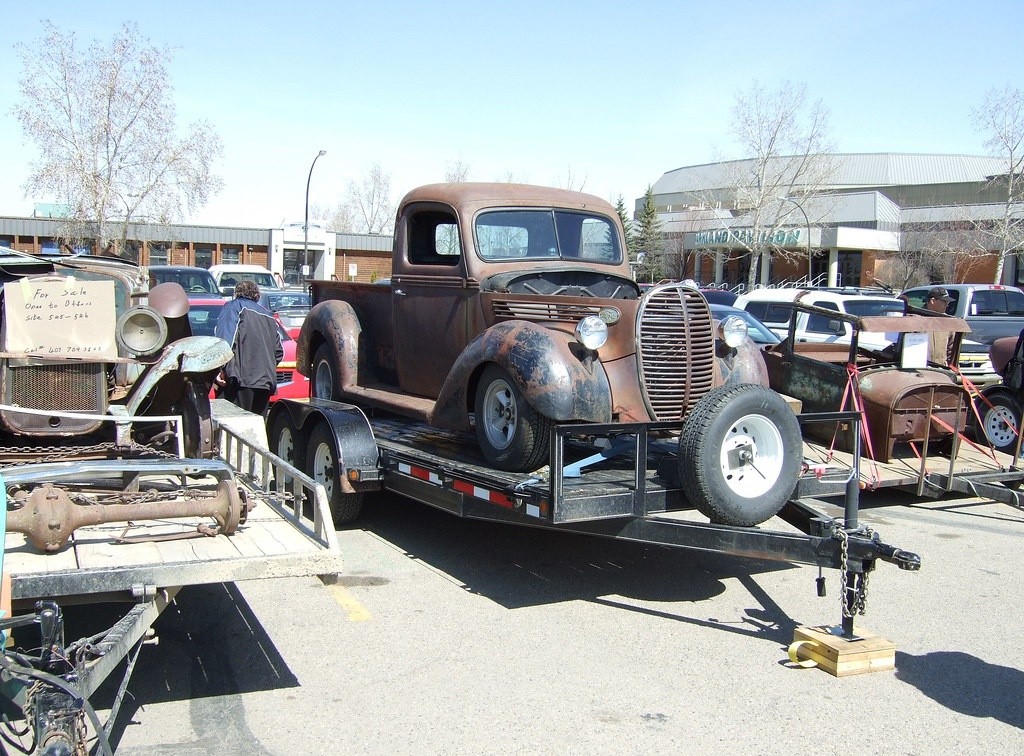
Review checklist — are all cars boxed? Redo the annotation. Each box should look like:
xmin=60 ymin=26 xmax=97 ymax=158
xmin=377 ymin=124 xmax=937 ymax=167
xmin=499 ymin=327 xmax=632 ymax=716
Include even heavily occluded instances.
xmin=0 ymin=180 xmax=1022 ymax=457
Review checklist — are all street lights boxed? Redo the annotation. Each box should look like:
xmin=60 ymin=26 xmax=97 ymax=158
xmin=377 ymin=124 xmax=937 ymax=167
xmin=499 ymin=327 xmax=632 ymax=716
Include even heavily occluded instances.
xmin=302 ymin=149 xmax=328 ymax=289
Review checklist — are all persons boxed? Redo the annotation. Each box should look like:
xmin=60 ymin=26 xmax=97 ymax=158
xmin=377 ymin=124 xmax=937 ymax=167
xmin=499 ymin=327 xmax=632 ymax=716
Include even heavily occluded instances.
xmin=921 ymin=287 xmax=955 ymax=363
xmin=214 ymin=281 xmax=285 ymax=414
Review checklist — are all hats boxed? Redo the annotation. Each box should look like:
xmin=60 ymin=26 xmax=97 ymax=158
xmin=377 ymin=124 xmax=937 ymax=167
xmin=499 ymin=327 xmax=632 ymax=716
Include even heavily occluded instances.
xmin=926 ymin=287 xmax=956 ymax=301
